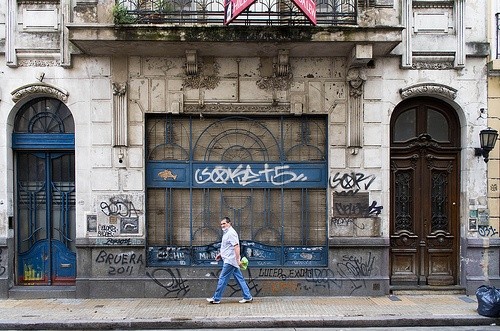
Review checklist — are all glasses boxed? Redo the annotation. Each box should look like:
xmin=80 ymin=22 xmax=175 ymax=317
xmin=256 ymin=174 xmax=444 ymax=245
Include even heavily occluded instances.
xmin=220 ymin=223 xmax=227 ymax=225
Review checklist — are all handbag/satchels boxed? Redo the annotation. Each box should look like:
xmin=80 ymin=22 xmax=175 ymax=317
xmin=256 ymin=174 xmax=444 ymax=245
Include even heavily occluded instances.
xmin=239 ymin=257 xmax=248 ymax=270
xmin=476 ymin=285 xmax=500 ymax=317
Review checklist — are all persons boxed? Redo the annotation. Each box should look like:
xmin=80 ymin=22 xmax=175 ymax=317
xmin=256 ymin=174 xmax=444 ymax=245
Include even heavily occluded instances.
xmin=206 ymin=216 xmax=254 ymax=303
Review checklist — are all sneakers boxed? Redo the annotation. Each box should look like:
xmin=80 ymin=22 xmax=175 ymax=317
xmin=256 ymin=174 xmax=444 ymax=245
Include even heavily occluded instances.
xmin=238 ymin=295 xmax=253 ymax=303
xmin=206 ymin=297 xmax=220 ymax=303
